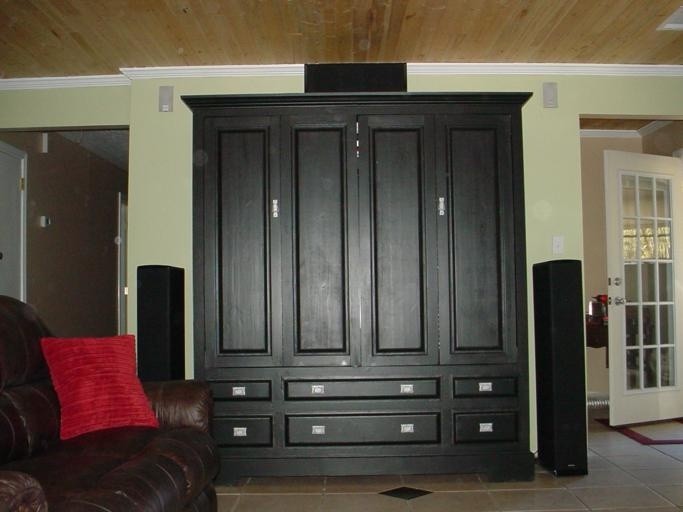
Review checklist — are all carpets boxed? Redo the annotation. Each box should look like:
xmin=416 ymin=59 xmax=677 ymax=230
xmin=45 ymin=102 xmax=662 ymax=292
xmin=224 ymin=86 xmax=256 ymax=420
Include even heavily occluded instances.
xmin=595 ymin=417 xmax=683 ymax=446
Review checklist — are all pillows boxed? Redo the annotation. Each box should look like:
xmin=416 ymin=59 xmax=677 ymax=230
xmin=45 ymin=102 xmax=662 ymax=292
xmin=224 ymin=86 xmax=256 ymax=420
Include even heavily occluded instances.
xmin=39 ymin=335 xmax=158 ymax=441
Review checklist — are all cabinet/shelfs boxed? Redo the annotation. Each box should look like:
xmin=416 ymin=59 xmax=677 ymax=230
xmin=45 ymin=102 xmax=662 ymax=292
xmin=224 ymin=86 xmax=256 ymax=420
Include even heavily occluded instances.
xmin=195 ymin=369 xmax=535 ymax=485
xmin=180 ymin=92 xmax=359 ymax=367
xmin=358 ymin=92 xmax=532 ymax=365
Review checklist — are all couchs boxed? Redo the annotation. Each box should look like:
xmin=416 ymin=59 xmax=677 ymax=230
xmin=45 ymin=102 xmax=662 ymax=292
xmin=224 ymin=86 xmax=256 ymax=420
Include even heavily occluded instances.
xmin=1 ymin=296 xmax=217 ymax=512
